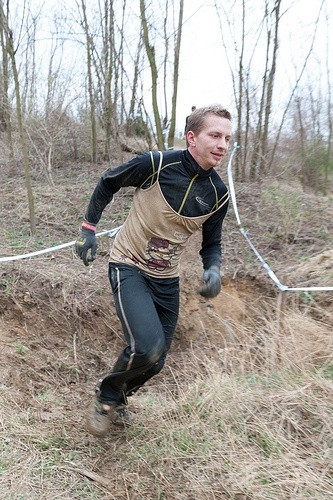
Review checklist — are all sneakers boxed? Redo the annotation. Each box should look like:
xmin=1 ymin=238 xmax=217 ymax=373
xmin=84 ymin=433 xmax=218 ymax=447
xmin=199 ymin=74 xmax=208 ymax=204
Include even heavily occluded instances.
xmin=86 ymin=375 xmax=115 ymax=437
xmin=112 ymin=403 xmax=136 ymax=426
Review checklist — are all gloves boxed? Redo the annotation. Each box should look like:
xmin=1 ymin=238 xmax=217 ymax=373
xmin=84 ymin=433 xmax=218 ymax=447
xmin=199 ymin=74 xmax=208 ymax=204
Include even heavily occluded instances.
xmin=75 ymin=222 xmax=98 ymax=267
xmin=196 ymin=265 xmax=220 ymax=298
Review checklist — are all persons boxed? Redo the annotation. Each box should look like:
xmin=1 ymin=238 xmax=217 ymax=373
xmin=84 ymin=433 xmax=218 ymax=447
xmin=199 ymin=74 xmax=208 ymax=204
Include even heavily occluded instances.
xmin=71 ymin=106 xmax=233 ymax=437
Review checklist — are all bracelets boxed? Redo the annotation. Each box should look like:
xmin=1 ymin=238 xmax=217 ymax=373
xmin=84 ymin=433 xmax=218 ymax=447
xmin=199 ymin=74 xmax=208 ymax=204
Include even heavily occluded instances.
xmin=81 ymin=222 xmax=96 ymax=231
